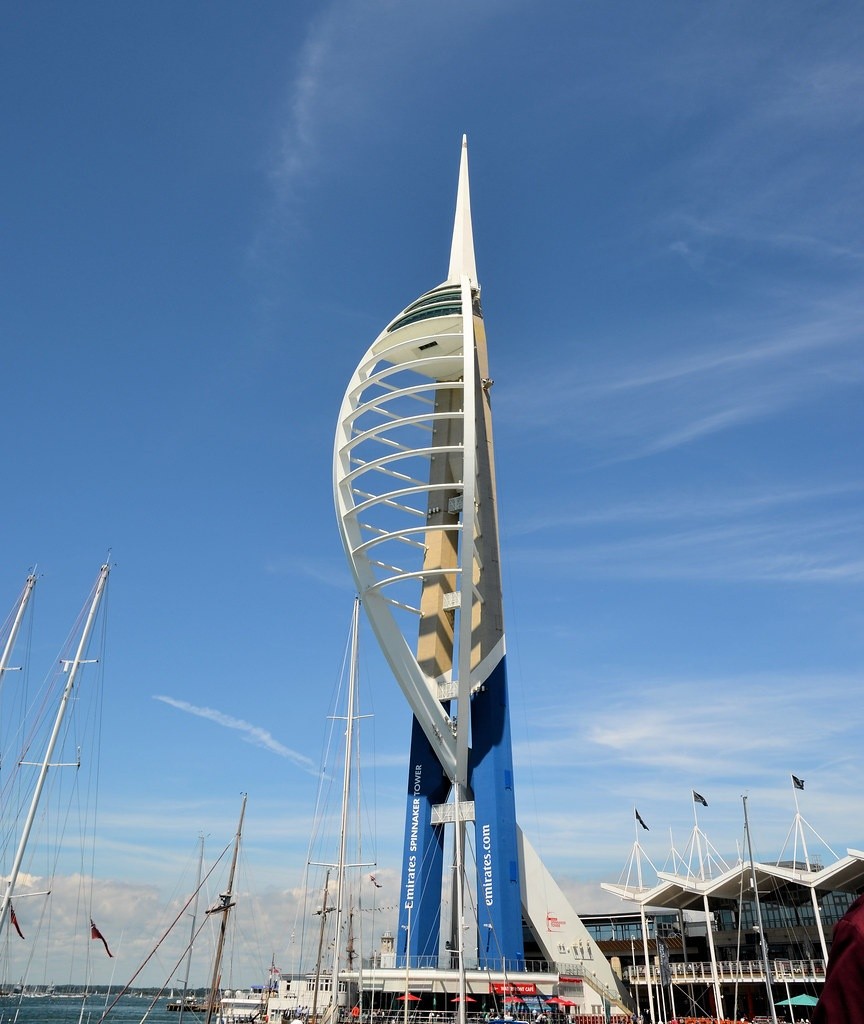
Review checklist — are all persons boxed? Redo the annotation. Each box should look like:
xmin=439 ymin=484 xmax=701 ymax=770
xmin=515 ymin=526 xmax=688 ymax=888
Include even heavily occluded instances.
xmin=808 ymin=896 xmax=864 ymax=1024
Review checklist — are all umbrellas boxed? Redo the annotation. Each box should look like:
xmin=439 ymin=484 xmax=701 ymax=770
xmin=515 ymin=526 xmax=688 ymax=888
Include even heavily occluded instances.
xmin=397 ymin=994 xmax=421 ymax=1002
xmin=774 ymin=995 xmax=818 ymax=1018
xmin=451 ymin=996 xmax=477 ymax=1004
xmin=563 ymin=1001 xmax=577 ymax=1007
xmin=544 ymin=997 xmax=567 ymax=1005
xmin=501 ymin=994 xmax=525 ymax=1004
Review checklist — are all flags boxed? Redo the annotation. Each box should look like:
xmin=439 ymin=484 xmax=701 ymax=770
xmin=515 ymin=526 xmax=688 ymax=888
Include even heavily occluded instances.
xmin=635 ymin=808 xmax=649 ymax=831
xmin=792 ymin=775 xmax=805 ymax=790
xmin=692 ymin=791 xmax=709 ymax=807
xmin=89 ymin=918 xmax=113 ymax=958
xmin=10 ymin=904 xmax=25 ymax=940
